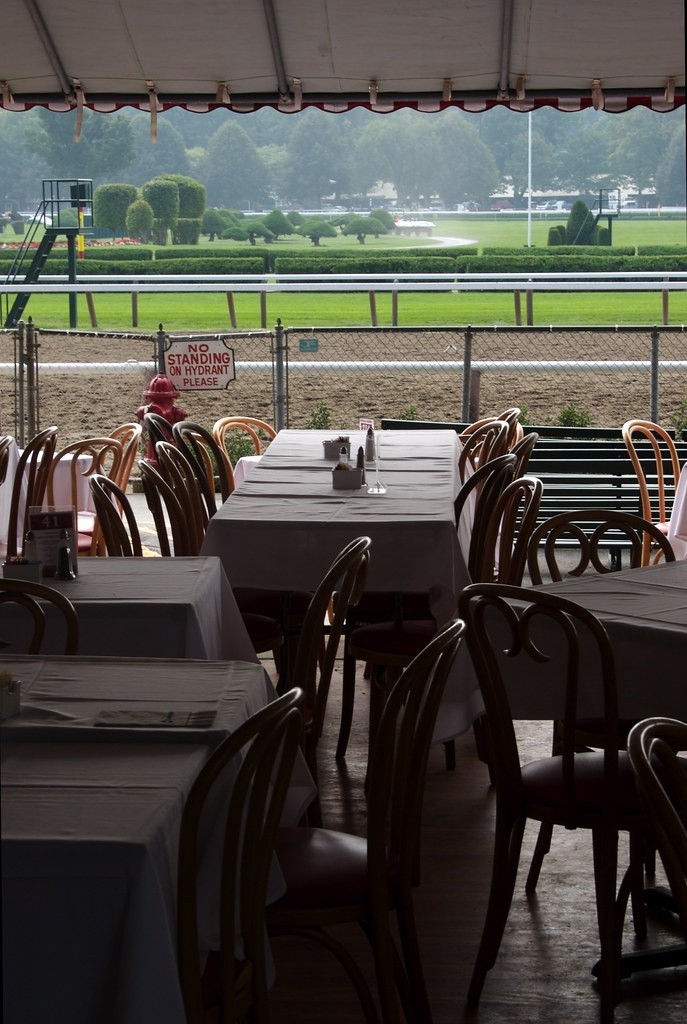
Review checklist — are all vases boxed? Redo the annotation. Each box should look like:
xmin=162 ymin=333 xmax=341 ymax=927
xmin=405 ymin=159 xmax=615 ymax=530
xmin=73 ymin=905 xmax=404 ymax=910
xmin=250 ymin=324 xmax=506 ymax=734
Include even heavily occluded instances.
xmin=133 ymin=481 xmax=145 ymax=493
xmin=128 ymin=477 xmax=143 ymax=486
xmin=182 ymin=476 xmax=201 ymax=492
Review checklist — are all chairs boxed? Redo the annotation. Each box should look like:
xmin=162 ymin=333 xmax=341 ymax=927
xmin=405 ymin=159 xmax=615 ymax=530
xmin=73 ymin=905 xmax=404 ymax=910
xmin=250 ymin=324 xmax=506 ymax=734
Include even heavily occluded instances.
xmin=0 ymin=408 xmax=687 ymax=1024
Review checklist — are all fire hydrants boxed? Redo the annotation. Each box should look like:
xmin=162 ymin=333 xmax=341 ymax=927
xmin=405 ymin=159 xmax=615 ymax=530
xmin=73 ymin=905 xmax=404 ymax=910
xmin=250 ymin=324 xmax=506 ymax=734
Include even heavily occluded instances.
xmin=133 ymin=372 xmax=189 ymax=477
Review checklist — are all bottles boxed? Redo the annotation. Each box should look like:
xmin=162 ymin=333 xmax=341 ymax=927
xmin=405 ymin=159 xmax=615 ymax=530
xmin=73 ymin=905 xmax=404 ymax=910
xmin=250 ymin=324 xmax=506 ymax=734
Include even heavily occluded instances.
xmin=356 ymin=446 xmax=367 ymax=486
xmin=331 ymin=447 xmax=352 ymax=471
xmin=364 ymin=428 xmax=377 ymax=465
xmin=24 ymin=531 xmax=37 ymax=562
xmin=54 ymin=531 xmax=77 ymax=581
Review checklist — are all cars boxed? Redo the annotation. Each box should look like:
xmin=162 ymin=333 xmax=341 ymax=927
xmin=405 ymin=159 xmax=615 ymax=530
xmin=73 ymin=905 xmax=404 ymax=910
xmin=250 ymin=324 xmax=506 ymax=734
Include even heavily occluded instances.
xmin=536 ymin=200 xmax=567 ymax=211
xmin=429 ymin=201 xmax=444 ymax=211
xmin=491 ymin=200 xmax=514 ymax=210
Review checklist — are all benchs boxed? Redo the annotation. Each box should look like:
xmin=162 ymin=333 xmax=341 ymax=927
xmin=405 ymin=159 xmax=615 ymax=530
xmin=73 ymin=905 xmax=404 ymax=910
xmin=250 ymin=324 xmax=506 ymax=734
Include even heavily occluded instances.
xmin=382 ymin=417 xmax=687 ymax=573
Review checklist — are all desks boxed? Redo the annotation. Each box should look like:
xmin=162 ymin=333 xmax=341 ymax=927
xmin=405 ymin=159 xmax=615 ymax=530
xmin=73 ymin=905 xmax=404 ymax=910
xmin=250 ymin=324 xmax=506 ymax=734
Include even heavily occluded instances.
xmin=0 ymin=654 xmax=318 ymax=831
xmin=0 ymin=554 xmax=265 ymax=662
xmin=194 ymin=427 xmax=481 ymax=719
xmin=18 ymin=447 xmax=124 ymax=556
xmin=451 ymin=558 xmax=687 ymax=992
xmin=0 ymin=738 xmax=277 ymax=1024
